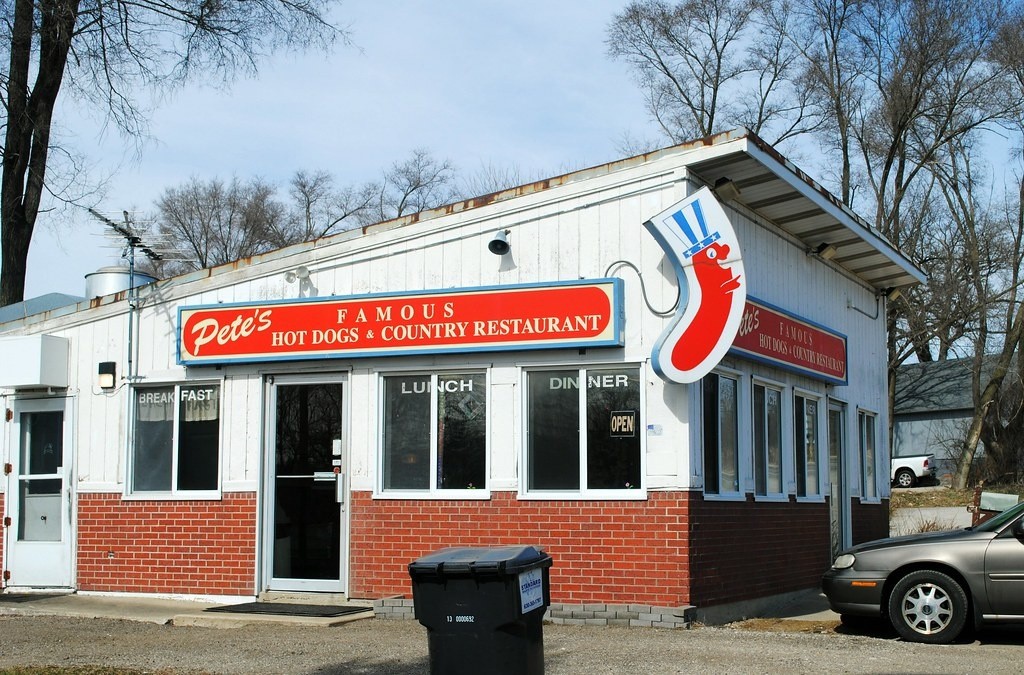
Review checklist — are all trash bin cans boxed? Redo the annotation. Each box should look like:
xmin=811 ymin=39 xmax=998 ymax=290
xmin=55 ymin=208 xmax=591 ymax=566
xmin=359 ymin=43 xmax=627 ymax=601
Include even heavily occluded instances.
xmin=405 ymin=541 xmax=555 ymax=675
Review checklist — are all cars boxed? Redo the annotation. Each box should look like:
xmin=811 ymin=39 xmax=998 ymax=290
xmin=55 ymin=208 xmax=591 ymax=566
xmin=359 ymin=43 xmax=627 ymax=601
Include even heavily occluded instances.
xmin=819 ymin=500 xmax=1024 ymax=644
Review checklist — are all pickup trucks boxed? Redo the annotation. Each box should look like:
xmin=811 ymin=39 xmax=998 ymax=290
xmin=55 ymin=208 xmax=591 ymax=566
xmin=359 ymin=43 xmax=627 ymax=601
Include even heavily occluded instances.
xmin=891 ymin=454 xmax=936 ymax=488
